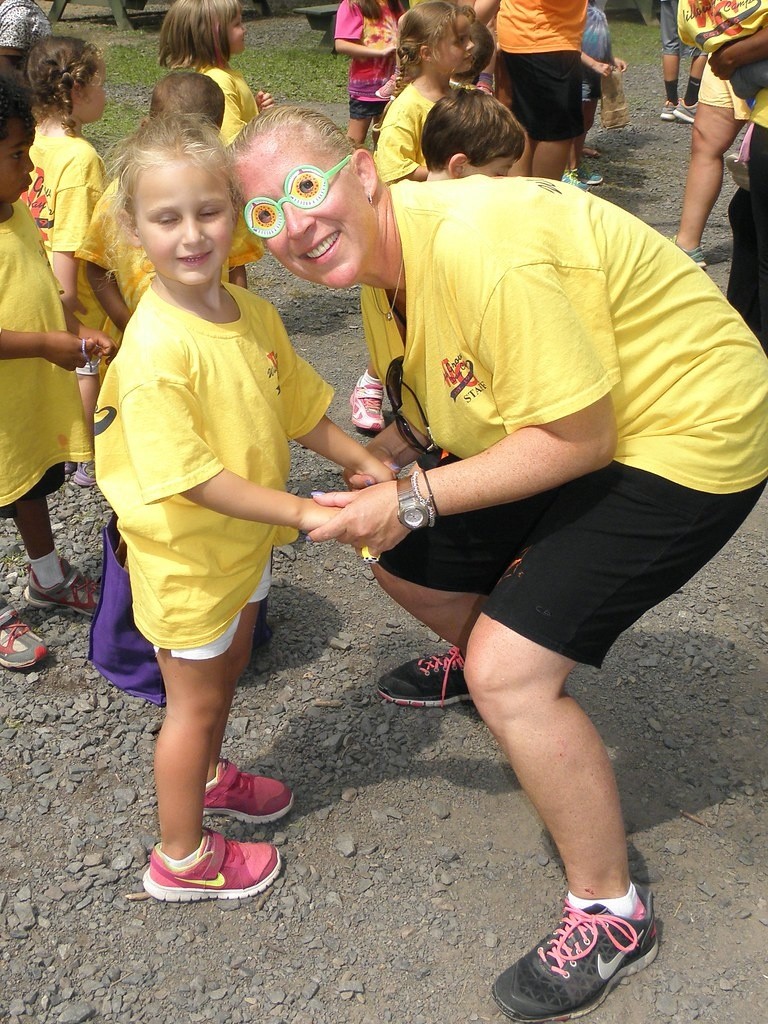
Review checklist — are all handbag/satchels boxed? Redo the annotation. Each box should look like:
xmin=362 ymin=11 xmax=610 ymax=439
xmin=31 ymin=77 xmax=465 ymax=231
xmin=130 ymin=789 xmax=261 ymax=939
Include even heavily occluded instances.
xmin=86 ymin=513 xmax=272 ymax=707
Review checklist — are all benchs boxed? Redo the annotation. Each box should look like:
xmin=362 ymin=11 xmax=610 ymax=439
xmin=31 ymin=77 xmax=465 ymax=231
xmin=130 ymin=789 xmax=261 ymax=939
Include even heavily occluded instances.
xmin=291 ymin=3 xmax=341 ymax=56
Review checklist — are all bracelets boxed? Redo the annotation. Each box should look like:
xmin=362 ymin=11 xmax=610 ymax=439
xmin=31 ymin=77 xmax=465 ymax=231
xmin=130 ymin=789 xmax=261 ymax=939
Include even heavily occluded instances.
xmin=421 ymin=469 xmax=440 ymax=519
xmin=411 ymin=470 xmax=435 ymax=528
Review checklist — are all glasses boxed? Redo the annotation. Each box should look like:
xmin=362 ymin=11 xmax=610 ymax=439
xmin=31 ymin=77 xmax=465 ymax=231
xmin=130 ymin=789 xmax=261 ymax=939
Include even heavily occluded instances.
xmin=243 ymin=155 xmax=351 ymax=239
xmin=385 ymin=356 xmax=436 ymax=456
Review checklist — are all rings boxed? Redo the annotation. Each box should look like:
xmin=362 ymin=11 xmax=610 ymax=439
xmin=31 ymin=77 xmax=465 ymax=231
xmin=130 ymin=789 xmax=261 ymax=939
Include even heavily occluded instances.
xmin=361 ymin=546 xmax=380 ymax=564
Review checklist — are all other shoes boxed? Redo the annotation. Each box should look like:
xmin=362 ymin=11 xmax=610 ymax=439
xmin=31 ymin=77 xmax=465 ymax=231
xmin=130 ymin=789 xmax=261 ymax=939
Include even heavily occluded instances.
xmin=74 ymin=461 xmax=96 ymax=486
xmin=725 ymin=154 xmax=750 ymax=191
xmin=582 ymin=145 xmax=601 ymax=159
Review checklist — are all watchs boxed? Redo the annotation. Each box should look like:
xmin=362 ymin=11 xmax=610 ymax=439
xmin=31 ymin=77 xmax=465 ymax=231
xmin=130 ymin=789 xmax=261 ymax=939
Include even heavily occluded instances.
xmin=397 ymin=470 xmax=435 ymax=530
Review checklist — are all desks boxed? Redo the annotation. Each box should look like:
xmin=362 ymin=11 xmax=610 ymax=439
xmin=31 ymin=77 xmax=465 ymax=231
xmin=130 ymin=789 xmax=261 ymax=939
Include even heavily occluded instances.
xmin=43 ymin=0 xmax=273 ymax=31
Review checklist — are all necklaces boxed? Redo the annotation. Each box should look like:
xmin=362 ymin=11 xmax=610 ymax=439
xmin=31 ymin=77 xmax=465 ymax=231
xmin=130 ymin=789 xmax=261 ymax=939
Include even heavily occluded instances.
xmin=371 ymin=286 xmax=404 ymax=321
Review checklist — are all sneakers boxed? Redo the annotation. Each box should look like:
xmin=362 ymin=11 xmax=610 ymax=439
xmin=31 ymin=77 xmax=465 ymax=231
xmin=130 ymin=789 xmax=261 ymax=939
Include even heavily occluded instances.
xmin=350 ymin=375 xmax=385 ymax=431
xmin=0 ymin=601 xmax=46 ymax=670
xmin=204 ymin=759 xmax=295 ymax=825
xmin=142 ymin=828 xmax=281 ymax=903
xmin=562 ymin=168 xmax=604 ymax=191
xmin=377 ymin=649 xmax=473 ymax=708
xmin=375 ymin=75 xmax=398 ymax=99
xmin=23 ymin=558 xmax=101 ymax=617
xmin=666 ymin=234 xmax=707 ymax=268
xmin=490 ymin=884 xmax=660 ymax=1023
xmin=660 ymin=98 xmax=698 ymax=123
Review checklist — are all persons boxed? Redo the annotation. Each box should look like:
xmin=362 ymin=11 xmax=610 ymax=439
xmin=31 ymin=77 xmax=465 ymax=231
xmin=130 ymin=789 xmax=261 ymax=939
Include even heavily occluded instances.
xmin=93 ymin=111 xmax=396 ymax=902
xmin=349 ymin=2 xmax=478 ymax=431
xmin=224 ymin=105 xmax=768 ymax=1023
xmin=660 ymin=0 xmax=768 ymax=356
xmin=0 ymin=0 xmax=275 ymax=669
xmin=334 ymin=0 xmax=627 ymax=192
